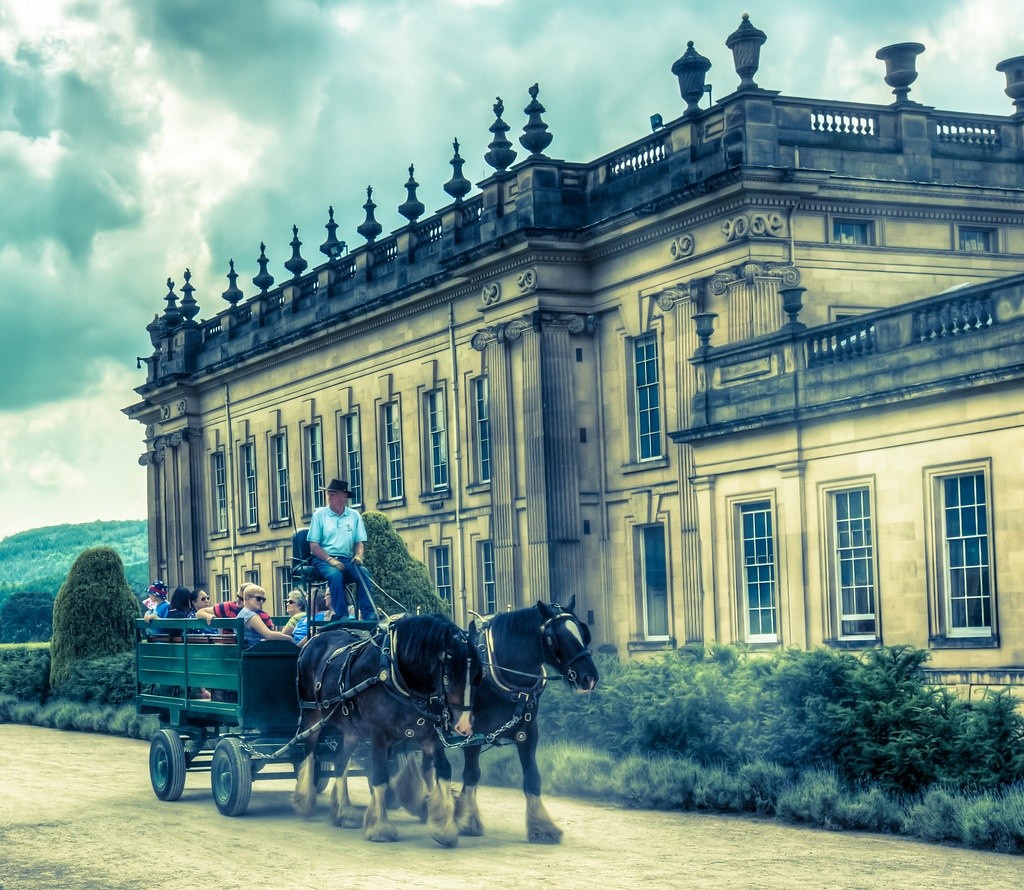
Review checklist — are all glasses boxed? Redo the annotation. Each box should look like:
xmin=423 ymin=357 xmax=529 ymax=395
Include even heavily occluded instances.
xmin=250 ymin=595 xmax=266 ymax=603
xmin=197 ymin=596 xmax=209 ymax=601
xmin=287 ymin=600 xmax=296 ymax=604
xmin=237 ymin=594 xmax=243 ymax=601
xmin=324 ymin=594 xmax=330 ymax=596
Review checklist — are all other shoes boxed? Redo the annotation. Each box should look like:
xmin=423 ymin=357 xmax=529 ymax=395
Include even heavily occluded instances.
xmin=339 ymin=616 xmax=349 ymax=621
xmin=368 ymin=614 xmax=376 ymax=621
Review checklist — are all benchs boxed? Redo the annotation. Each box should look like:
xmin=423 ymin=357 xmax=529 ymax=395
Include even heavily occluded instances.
xmin=132 ymin=617 xmax=316 ymax=711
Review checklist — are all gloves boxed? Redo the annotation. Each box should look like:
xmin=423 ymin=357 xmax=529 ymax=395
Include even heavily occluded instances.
xmin=351 ymin=554 xmax=364 ymax=566
xmin=327 ymin=557 xmax=344 ymax=570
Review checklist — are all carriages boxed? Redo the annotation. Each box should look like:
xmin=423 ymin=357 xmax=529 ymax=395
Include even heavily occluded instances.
xmin=127 ymin=527 xmax=600 ymax=848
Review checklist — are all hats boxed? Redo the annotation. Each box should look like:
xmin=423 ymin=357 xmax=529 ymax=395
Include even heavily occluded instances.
xmin=142 ymin=598 xmax=171 ymax=610
xmin=318 ymin=479 xmax=355 ymax=498
xmin=144 ymin=580 xmax=168 ymax=599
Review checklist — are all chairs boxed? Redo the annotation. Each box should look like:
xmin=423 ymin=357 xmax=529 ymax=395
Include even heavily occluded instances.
xmin=291 ymin=529 xmax=358 ymax=621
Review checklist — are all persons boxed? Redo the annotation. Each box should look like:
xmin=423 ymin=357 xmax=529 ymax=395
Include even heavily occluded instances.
xmin=235 ymin=584 xmax=295 ymax=648
xmin=195 ymin=583 xmax=274 ymax=633
xmin=144 ymin=581 xmax=170 ymax=634
xmin=293 ymin=587 xmax=355 ymax=648
xmin=282 ymin=590 xmax=308 ymax=635
xmin=307 ymin=479 xmax=382 ymax=615
xmin=187 ymin=589 xmax=219 ymax=634
xmin=167 ymin=588 xmax=191 ymax=636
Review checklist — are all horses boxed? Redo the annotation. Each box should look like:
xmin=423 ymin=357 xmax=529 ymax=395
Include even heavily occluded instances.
xmin=293 ymin=613 xmax=483 ymax=847
xmin=392 ymin=593 xmax=600 ymax=844
